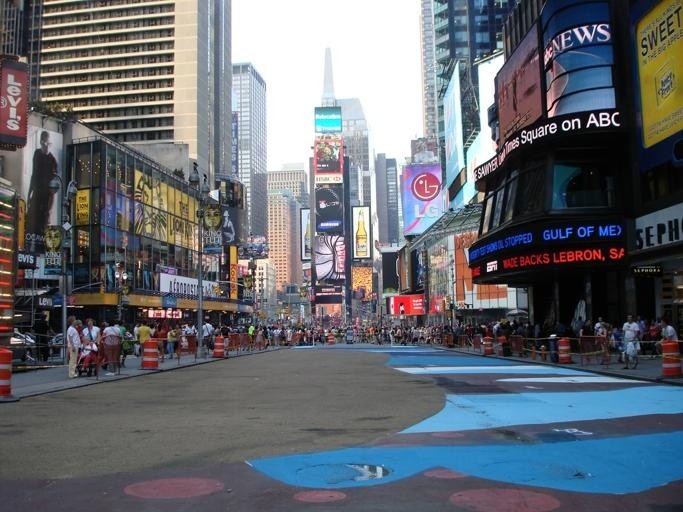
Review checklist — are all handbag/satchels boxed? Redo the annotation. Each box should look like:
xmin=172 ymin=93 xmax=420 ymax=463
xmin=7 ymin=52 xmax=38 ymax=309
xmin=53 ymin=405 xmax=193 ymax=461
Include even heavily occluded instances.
xmin=207 ymin=342 xmax=214 ymax=350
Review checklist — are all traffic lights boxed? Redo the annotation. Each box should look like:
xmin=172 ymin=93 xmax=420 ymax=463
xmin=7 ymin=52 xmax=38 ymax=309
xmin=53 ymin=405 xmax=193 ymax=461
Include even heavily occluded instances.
xmin=446 ymin=297 xmax=452 ymax=303
xmin=439 ymin=301 xmax=444 ymax=312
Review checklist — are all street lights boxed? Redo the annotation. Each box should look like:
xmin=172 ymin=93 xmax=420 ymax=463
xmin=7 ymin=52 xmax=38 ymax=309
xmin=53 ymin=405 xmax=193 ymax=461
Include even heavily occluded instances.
xmin=186 ymin=161 xmax=211 ymax=360
xmin=113 ymin=262 xmax=128 ymax=320
xmin=47 ymin=172 xmax=81 ymax=367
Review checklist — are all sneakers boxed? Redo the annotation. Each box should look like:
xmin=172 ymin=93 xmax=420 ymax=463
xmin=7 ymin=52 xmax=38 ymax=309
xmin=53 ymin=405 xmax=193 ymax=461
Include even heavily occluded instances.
xmin=105 ymin=372 xmax=114 ymax=376
xmin=621 ymin=367 xmax=629 ymax=370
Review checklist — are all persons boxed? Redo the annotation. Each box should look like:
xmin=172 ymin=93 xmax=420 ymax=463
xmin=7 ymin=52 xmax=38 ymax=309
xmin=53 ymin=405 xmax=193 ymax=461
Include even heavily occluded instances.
xmin=222 ymin=208 xmax=236 ymax=243
xmin=204 ymin=208 xmax=221 ymax=231
xmin=23 ymin=129 xmax=58 ymax=251
xmin=33 ymin=314 xmax=57 ymax=360
xmin=42 ymin=225 xmax=62 ymax=252
xmin=372 ymin=290 xmax=377 ymax=301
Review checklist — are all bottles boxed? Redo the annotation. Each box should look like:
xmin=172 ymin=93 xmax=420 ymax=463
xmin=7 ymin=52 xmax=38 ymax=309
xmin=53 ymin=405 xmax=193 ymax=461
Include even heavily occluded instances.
xmin=304 ymin=215 xmax=310 ymax=257
xmin=356 ymin=212 xmax=367 ymax=257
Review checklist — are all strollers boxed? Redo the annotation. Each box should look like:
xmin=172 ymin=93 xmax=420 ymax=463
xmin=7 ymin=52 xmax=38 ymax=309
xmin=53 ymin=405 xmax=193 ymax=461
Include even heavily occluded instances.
xmin=75 ymin=340 xmax=99 ymax=378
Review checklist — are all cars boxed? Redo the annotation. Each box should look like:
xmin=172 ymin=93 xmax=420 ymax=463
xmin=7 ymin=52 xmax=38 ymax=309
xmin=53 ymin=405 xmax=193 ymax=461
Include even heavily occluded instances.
xmin=10 ymin=330 xmax=39 ymax=361
